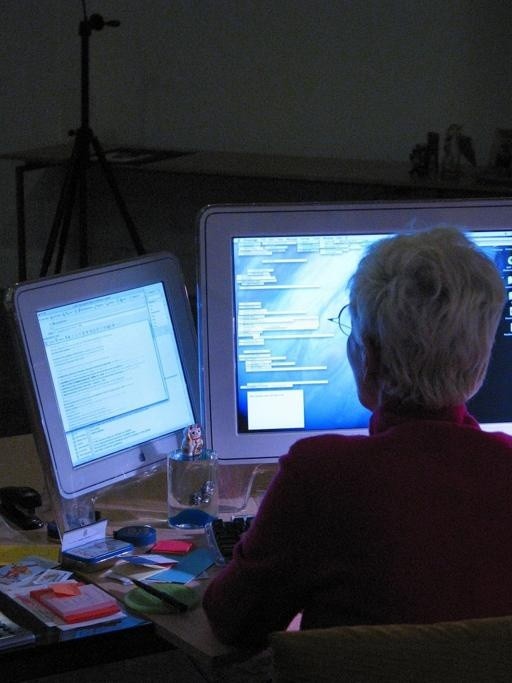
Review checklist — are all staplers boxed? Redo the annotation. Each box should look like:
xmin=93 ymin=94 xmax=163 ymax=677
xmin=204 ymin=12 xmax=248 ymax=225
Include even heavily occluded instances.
xmin=0 ymin=486 xmax=44 ymax=531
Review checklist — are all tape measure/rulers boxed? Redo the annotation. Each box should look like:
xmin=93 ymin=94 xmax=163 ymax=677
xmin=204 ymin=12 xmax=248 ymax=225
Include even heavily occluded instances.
xmin=112 ymin=525 xmax=157 ymax=546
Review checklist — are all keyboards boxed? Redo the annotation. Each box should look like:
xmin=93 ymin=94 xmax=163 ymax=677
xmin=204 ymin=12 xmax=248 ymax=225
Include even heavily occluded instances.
xmin=203 ymin=515 xmax=255 ymax=569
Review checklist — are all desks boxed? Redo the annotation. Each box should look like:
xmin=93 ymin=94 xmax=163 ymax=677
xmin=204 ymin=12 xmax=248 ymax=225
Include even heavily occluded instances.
xmin=1 ymin=428 xmax=283 ymax=681
xmin=1 ymin=135 xmax=512 ymax=281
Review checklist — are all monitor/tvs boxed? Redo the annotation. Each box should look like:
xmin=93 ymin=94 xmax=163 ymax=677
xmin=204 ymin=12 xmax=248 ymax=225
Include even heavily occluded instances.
xmin=196 ymin=195 xmax=512 ymax=468
xmin=0 ymin=248 xmax=200 ymax=581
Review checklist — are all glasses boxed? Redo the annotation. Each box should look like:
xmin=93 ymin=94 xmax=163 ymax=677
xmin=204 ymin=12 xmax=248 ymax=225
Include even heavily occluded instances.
xmin=328 ymin=302 xmax=358 ymax=336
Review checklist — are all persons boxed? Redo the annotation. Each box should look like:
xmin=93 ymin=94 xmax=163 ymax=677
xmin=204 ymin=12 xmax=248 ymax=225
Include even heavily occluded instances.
xmin=200 ymin=224 xmax=511 ymax=652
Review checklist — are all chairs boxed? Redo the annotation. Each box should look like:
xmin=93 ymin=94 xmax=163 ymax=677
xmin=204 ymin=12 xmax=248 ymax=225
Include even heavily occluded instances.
xmin=264 ymin=614 xmax=510 ymax=682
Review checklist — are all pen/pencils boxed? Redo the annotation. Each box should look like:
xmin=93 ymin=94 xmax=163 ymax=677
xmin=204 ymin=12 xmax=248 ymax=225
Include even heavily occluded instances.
xmin=128 ymin=576 xmax=188 ymax=613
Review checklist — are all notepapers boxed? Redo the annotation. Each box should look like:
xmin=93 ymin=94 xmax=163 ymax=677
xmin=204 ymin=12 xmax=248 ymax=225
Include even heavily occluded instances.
xmin=30 ymin=581 xmax=122 ymax=625
xmin=151 ymin=539 xmax=193 ymax=555
